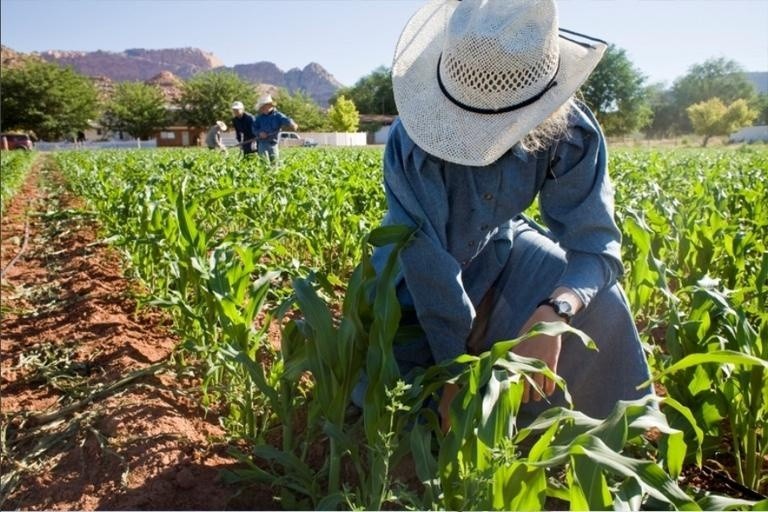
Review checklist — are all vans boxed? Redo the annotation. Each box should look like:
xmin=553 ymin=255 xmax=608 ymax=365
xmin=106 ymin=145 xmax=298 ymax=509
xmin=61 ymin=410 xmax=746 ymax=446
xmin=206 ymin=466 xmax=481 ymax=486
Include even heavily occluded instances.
xmin=0 ymin=134 xmax=33 ymax=152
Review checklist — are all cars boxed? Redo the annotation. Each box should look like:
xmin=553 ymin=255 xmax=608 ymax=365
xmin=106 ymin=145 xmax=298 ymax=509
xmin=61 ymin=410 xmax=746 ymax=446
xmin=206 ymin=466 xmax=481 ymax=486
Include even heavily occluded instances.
xmin=279 ymin=132 xmax=316 ymax=147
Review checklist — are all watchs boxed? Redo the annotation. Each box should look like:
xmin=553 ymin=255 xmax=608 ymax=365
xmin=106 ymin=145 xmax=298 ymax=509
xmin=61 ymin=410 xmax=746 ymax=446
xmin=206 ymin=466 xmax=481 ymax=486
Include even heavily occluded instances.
xmin=537 ymin=295 xmax=574 ymax=332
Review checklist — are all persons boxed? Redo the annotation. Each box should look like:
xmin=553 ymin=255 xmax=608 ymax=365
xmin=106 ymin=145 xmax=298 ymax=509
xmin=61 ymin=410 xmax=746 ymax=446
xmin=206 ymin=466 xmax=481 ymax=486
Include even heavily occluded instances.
xmin=345 ymin=0 xmax=655 ymax=484
xmin=251 ymin=95 xmax=300 ymax=166
xmin=228 ymin=100 xmax=257 ymax=155
xmin=203 ymin=119 xmax=228 ymax=155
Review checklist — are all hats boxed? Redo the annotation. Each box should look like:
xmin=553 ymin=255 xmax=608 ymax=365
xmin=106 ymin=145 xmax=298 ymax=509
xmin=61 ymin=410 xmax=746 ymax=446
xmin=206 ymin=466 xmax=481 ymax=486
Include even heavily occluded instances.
xmin=232 ymin=101 xmax=244 ymax=110
xmin=253 ymin=95 xmax=279 ymax=110
xmin=392 ymin=1 xmax=609 ymax=167
xmin=217 ymin=121 xmax=228 ymax=131
